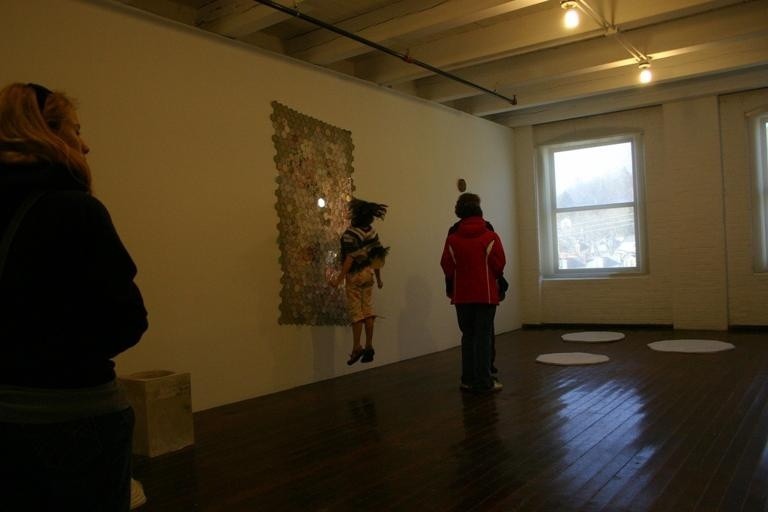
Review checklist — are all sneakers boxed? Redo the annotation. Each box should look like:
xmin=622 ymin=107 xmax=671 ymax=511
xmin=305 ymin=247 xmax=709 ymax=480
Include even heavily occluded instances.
xmin=347 ymin=347 xmax=375 ymax=366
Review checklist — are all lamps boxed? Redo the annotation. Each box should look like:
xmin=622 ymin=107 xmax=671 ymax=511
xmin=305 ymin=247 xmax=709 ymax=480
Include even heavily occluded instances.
xmin=559 ymin=0 xmax=652 ymax=85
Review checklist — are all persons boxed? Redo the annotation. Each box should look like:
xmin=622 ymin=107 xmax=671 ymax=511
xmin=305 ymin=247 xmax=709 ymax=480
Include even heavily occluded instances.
xmin=1 ymin=80 xmax=149 ymax=509
xmin=557 ymin=215 xmax=637 ymax=269
xmin=440 ymin=191 xmax=507 ymax=392
xmin=330 ymin=199 xmax=389 ymax=366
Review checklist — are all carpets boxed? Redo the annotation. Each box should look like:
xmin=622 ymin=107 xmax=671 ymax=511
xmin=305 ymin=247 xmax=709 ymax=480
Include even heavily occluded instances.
xmin=535 ymin=330 xmax=737 ymax=368
